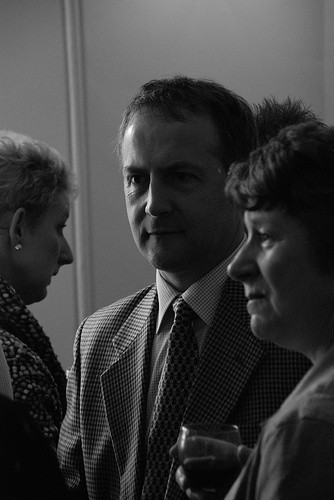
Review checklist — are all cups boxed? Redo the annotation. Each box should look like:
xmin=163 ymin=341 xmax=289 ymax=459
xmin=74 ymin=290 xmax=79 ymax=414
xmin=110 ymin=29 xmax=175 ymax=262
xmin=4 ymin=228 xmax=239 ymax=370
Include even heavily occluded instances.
xmin=174 ymin=423 xmax=243 ymax=500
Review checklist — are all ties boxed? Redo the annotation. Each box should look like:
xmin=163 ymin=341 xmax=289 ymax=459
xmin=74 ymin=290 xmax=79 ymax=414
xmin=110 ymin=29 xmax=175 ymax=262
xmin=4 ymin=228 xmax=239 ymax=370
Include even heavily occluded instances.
xmin=140 ymin=297 xmax=198 ymax=500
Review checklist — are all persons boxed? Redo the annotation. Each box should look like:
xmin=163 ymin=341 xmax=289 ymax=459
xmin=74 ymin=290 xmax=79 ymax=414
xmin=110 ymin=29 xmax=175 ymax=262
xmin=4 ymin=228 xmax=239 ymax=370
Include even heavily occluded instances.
xmin=169 ymin=120 xmax=334 ymax=500
xmin=56 ymin=78 xmax=313 ymax=500
xmin=0 ymin=130 xmax=74 ymax=500
